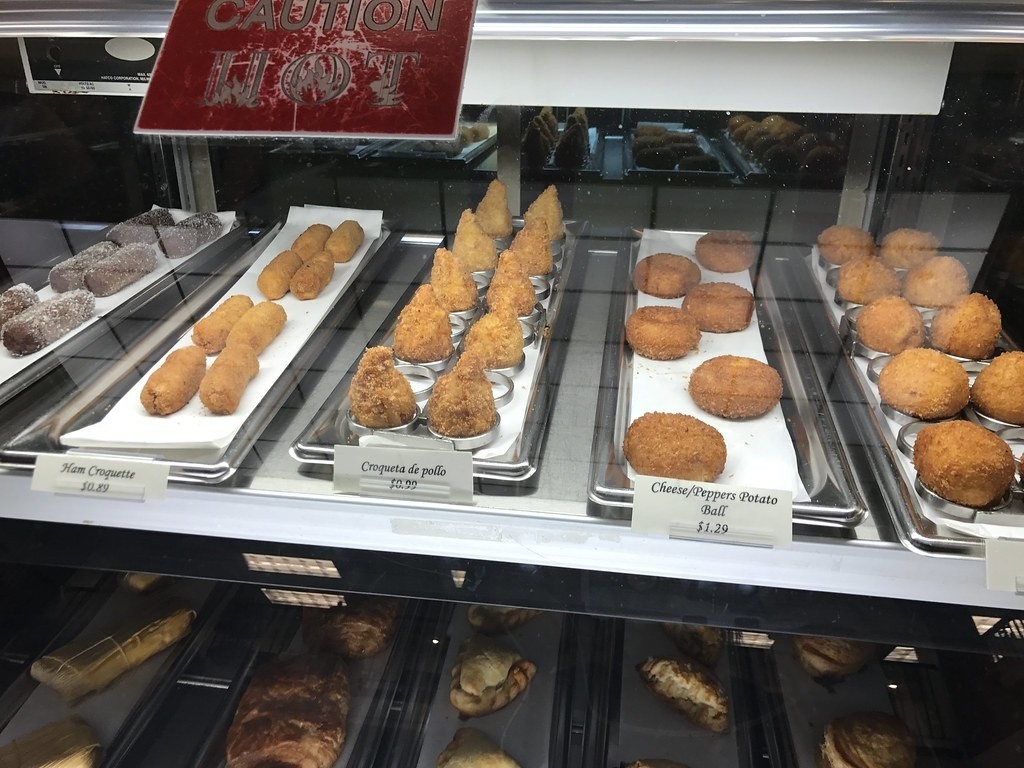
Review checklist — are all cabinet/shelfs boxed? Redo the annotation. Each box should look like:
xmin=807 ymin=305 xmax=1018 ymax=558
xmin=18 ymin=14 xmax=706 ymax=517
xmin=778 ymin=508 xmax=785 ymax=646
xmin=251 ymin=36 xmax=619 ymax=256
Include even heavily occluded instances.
xmin=0 ymin=1 xmax=1024 ymax=768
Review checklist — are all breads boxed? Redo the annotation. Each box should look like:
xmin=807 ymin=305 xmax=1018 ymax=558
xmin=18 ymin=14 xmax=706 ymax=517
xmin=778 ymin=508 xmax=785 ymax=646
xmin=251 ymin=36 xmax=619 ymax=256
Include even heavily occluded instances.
xmin=622 ymin=229 xmax=783 ymax=481
xmin=351 ymin=180 xmax=564 ymax=439
xmin=526 ymin=107 xmax=838 ymax=172
xmin=818 ymin=224 xmax=1024 ymax=509
xmin=138 ymin=220 xmax=365 ymax=416
xmin=0 ymin=209 xmax=220 ymax=356
xmin=216 ymin=567 xmax=924 ymax=768
